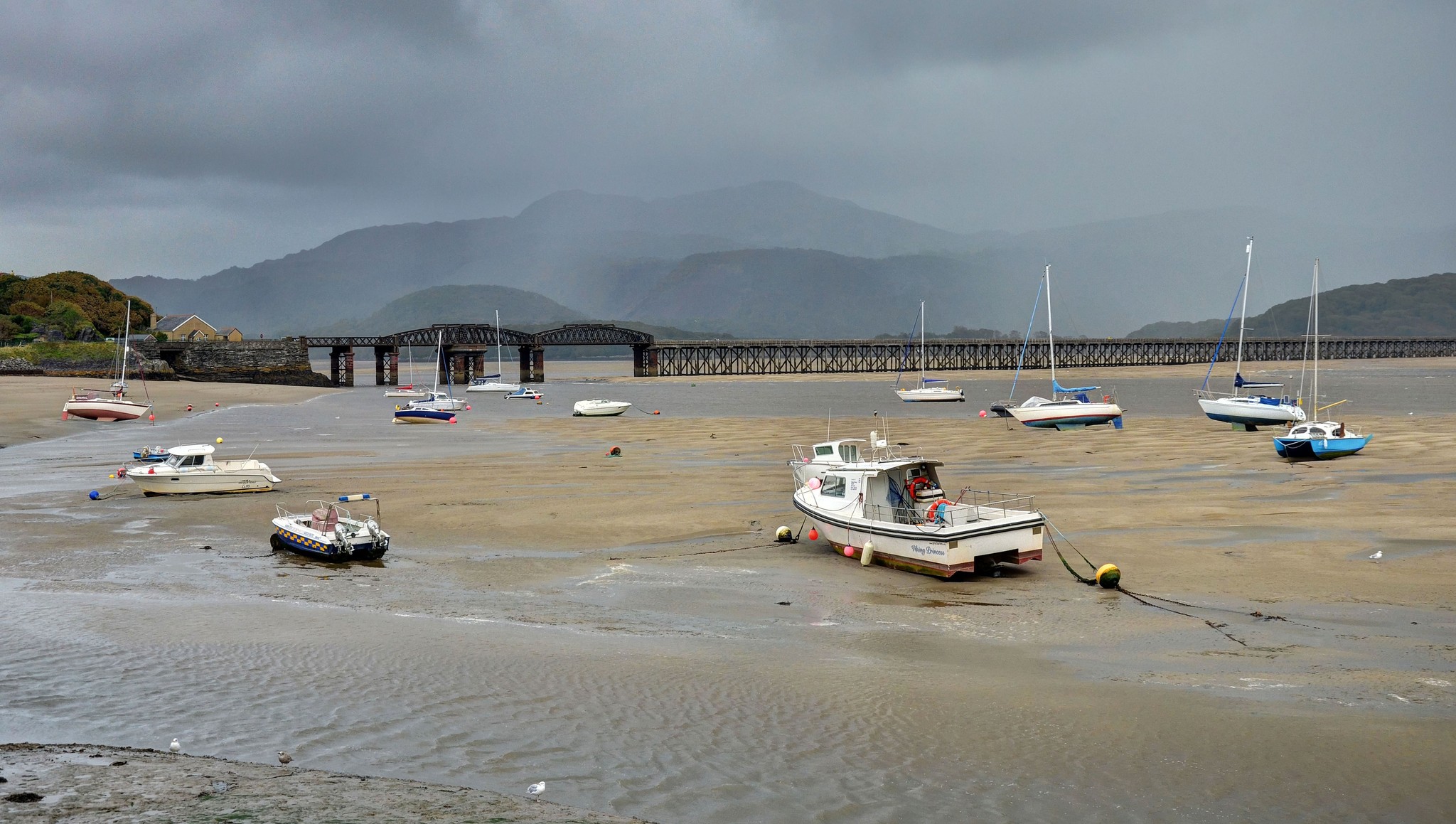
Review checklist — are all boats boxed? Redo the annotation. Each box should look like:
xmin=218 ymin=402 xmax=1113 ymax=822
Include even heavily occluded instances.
xmin=503 ymin=387 xmax=544 ymax=400
xmin=989 ymin=399 xmax=1020 ymax=418
xmin=464 ymin=380 xmax=488 ymax=388
xmin=132 ymin=445 xmax=169 ymax=462
xmin=786 ymin=408 xmax=1047 ymax=578
xmin=270 ymin=493 xmax=391 ymax=564
xmin=406 ymin=383 xmax=468 ymax=411
xmin=573 ymin=397 xmax=632 ymax=416
xmin=124 ymin=444 xmax=283 ymax=497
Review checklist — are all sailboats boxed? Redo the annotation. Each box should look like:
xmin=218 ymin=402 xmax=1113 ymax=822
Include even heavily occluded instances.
xmin=391 ymin=332 xmax=458 ymax=425
xmin=1271 ymin=256 xmax=1373 ymax=462
xmin=465 ymin=310 xmax=522 ymax=393
xmin=890 ymin=299 xmax=965 ymax=402
xmin=61 ymin=299 xmax=152 ymax=422
xmin=383 ymin=340 xmax=426 ymax=398
xmin=999 ymin=264 xmax=1128 ymax=431
xmin=110 ymin=327 xmax=128 ymax=397
xmin=1192 ymin=236 xmax=1306 ymax=432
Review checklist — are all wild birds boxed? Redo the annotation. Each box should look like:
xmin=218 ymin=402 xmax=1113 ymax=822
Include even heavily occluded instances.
xmin=525 ymin=781 xmax=546 ymax=802
xmin=1369 ymin=551 xmax=1383 ymax=564
xmin=278 ymin=752 xmax=294 ymax=770
xmin=169 ymin=738 xmax=182 ymax=759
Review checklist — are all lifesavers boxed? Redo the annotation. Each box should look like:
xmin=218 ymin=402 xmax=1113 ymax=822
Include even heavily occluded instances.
xmin=929 ymin=499 xmax=953 ymax=523
xmin=909 ymin=477 xmax=932 ymax=503
